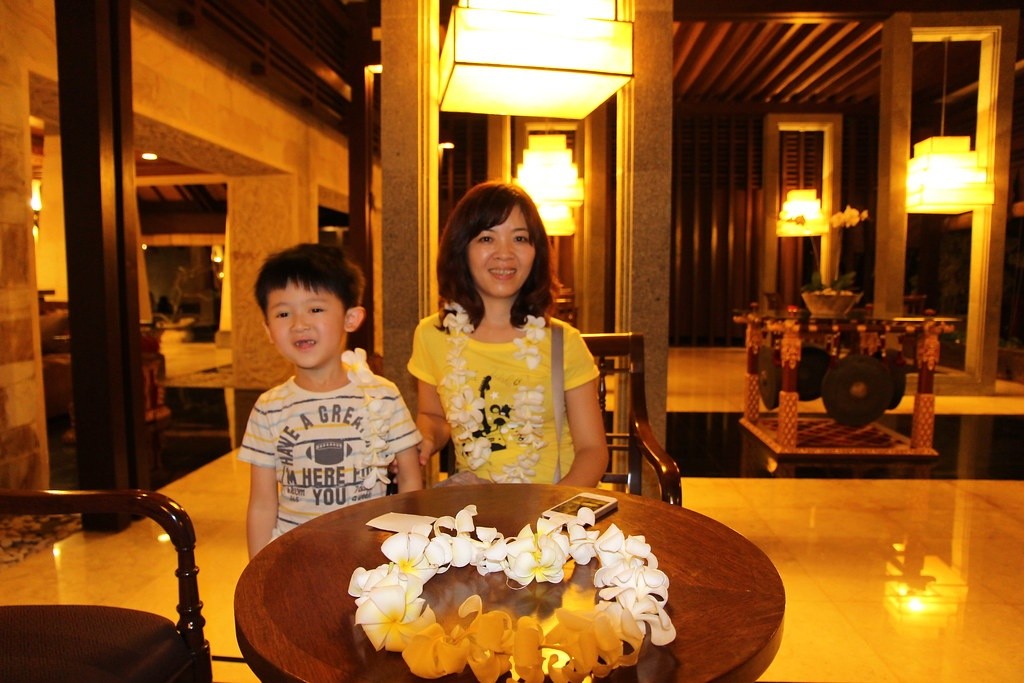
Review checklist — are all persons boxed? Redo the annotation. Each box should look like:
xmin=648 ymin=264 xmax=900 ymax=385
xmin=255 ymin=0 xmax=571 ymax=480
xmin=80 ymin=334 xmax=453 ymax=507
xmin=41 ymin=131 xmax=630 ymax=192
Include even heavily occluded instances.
xmin=407 ymin=180 xmax=609 ymax=488
xmin=237 ymin=244 xmax=424 ymax=561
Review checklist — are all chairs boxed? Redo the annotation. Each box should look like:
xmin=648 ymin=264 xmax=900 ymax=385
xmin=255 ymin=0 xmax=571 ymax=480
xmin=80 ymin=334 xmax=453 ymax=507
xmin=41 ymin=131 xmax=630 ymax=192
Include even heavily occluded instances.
xmin=0 ymin=488 xmax=213 ymax=683
xmin=438 ymin=332 xmax=682 ymax=507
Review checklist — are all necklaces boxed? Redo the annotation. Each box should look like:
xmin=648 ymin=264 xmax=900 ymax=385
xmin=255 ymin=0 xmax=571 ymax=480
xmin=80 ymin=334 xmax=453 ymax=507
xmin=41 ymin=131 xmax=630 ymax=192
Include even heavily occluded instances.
xmin=442 ymin=300 xmax=548 ymax=483
xmin=348 ymin=504 xmax=676 ymax=683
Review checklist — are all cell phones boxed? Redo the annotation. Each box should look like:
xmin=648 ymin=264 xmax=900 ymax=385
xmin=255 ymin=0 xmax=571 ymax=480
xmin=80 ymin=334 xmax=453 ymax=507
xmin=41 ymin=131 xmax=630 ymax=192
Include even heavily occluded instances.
xmin=541 ymin=493 xmax=618 ymax=528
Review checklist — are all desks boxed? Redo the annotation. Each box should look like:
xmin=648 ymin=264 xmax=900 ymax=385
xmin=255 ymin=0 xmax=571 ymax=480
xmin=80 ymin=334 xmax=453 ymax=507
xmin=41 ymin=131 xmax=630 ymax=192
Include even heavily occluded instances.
xmin=233 ymin=483 xmax=787 ymax=683
xmin=735 ymin=311 xmax=961 ymax=458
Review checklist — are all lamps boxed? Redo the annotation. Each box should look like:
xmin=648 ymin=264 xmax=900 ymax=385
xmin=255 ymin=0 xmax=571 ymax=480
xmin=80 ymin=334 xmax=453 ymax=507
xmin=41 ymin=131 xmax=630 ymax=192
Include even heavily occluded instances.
xmin=776 ymin=132 xmax=829 ymax=237
xmin=437 ymin=0 xmax=633 ymax=118
xmin=906 ymin=36 xmax=996 ymax=216
xmin=511 ymin=120 xmax=585 ymax=237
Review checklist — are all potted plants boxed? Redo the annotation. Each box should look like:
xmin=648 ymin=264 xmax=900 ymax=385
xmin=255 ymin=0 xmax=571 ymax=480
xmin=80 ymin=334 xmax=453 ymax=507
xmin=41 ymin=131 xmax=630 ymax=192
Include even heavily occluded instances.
xmin=802 ymin=272 xmax=865 ymax=318
